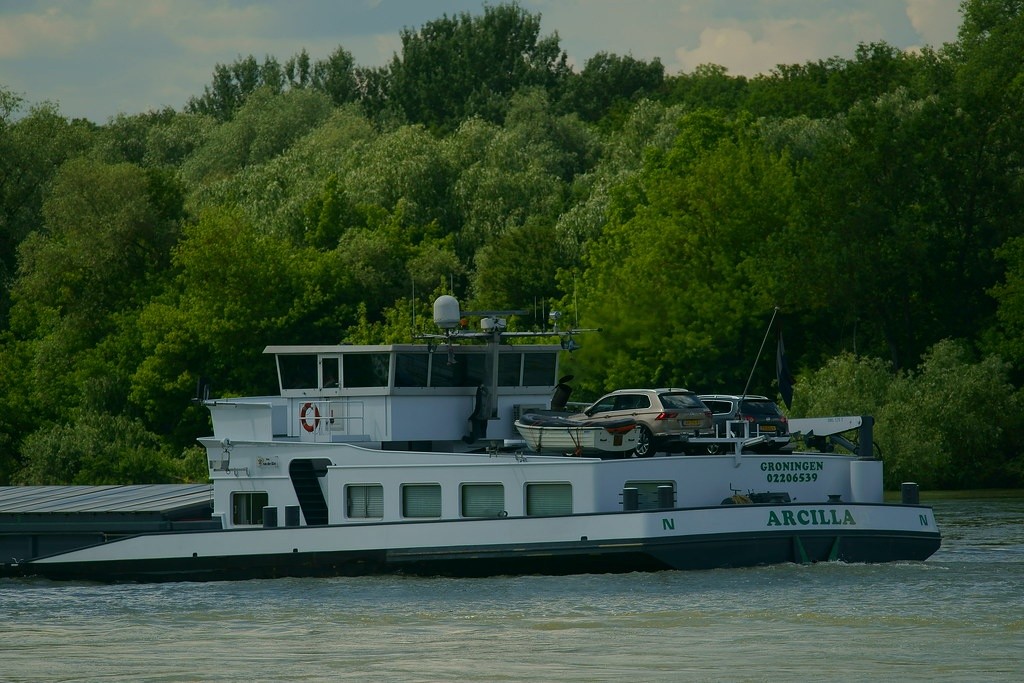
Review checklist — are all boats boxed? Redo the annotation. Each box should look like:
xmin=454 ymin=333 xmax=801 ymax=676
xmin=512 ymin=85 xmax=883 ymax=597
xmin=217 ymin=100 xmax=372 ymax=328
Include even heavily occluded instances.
xmin=27 ymin=294 xmax=943 ymax=582
xmin=514 ymin=414 xmax=640 ymax=458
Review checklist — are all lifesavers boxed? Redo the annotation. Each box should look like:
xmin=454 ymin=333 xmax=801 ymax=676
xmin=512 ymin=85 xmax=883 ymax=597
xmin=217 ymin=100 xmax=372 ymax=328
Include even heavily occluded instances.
xmin=301 ymin=403 xmax=320 ymax=432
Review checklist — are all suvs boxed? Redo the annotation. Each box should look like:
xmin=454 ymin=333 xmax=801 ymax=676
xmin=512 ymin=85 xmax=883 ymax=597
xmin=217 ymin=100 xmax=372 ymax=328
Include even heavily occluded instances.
xmin=697 ymin=394 xmax=791 ymax=456
xmin=569 ymin=386 xmax=713 ymax=458
